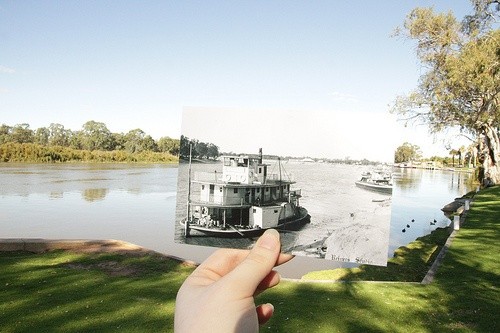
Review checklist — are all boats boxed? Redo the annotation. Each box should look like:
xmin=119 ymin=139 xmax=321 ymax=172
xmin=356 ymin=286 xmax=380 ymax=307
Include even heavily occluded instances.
xmin=394 ymin=160 xmax=435 ymax=171
xmin=355 ymin=165 xmax=392 ymax=194
xmin=179 ymin=142 xmax=308 ymax=241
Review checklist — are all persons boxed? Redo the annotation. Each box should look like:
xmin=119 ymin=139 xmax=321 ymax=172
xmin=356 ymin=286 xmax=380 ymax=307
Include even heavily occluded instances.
xmin=172 ymin=227 xmax=297 ymax=332
